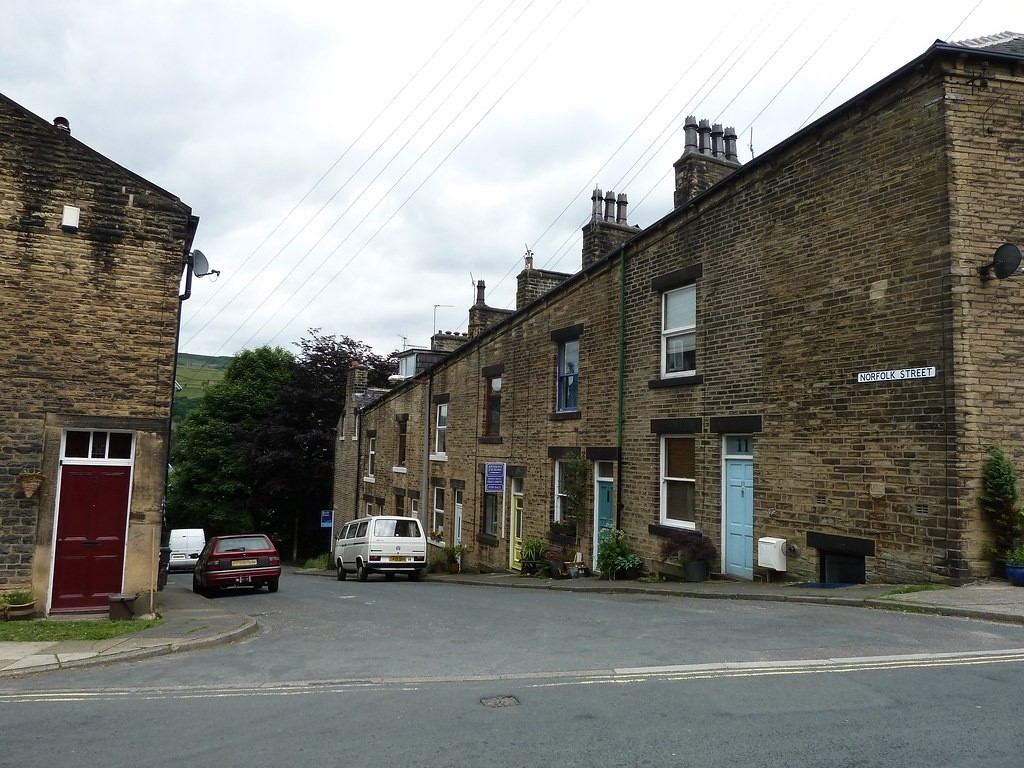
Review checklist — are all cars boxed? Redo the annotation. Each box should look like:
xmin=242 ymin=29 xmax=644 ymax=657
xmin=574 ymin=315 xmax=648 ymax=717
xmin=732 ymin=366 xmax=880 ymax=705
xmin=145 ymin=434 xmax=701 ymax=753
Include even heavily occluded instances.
xmin=192 ymin=534 xmax=281 ymax=595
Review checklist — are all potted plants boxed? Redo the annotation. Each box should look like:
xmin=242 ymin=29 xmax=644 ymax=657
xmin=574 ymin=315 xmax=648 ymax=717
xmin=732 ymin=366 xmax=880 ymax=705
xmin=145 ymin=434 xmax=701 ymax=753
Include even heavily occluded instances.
xmin=546 ymin=452 xmax=708 ymax=588
xmin=977 ymin=440 xmax=1024 ymax=587
xmin=430 ymin=550 xmax=446 ymax=574
xmin=2 ymin=585 xmax=38 ymax=620
xmin=18 ymin=468 xmax=47 ymax=500
xmin=519 ymin=536 xmax=546 ymax=576
xmin=442 ymin=544 xmax=466 ymax=574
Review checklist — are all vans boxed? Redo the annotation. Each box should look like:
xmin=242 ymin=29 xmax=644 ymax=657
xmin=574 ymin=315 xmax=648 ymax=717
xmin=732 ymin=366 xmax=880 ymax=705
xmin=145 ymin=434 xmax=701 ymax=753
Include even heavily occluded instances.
xmin=334 ymin=515 xmax=428 ymax=582
xmin=166 ymin=528 xmax=207 ymax=573
